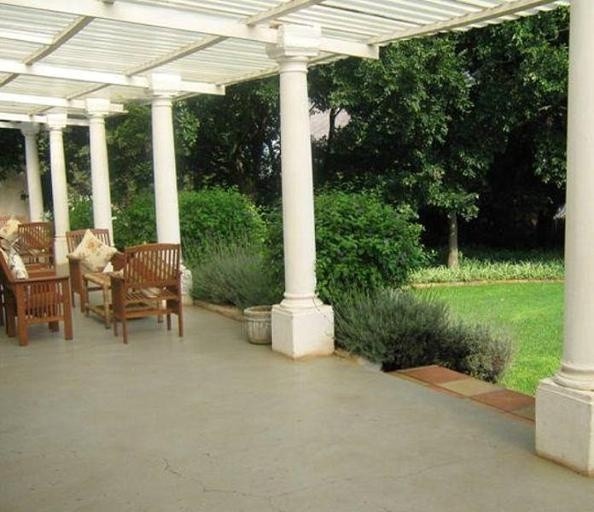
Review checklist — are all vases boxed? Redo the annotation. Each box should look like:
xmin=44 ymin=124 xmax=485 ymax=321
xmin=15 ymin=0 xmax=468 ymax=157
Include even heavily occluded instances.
xmin=243 ymin=304 xmax=272 ymax=344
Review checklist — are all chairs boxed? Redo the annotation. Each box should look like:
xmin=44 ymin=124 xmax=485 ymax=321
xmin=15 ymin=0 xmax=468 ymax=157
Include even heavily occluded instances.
xmin=0 ymin=214 xmax=184 ymax=349
